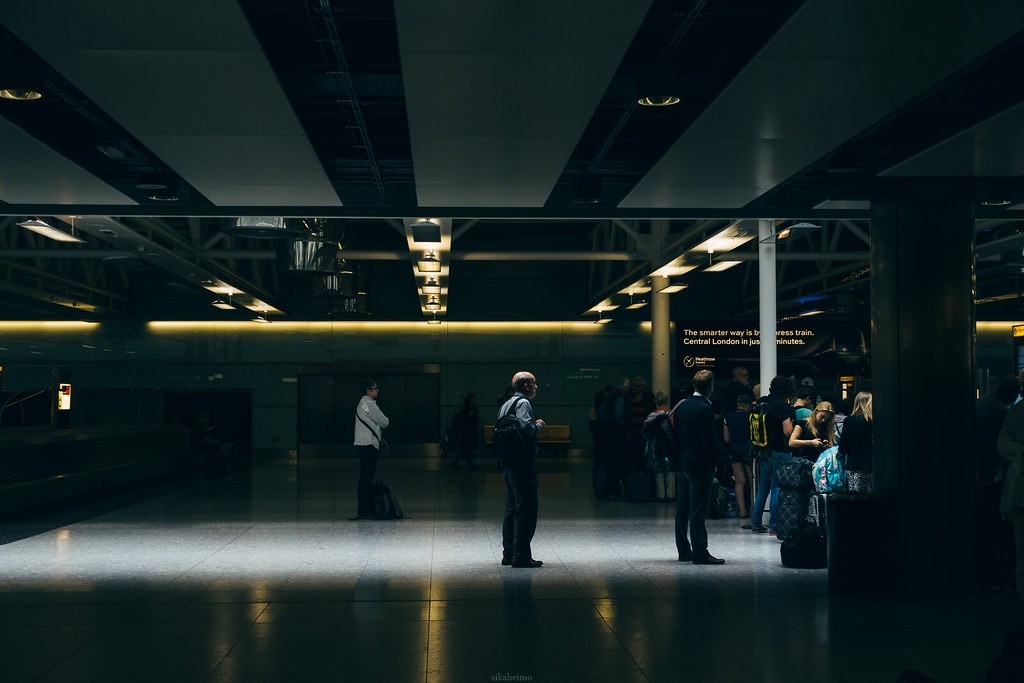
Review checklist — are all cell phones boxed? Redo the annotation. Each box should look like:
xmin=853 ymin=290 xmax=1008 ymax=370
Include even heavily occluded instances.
xmin=820 ymin=442 xmax=829 ymax=445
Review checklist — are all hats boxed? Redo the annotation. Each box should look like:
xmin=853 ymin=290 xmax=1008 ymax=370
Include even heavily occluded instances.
xmin=802 ymin=377 xmax=813 ymax=387
xmin=736 ymin=394 xmax=752 ymax=405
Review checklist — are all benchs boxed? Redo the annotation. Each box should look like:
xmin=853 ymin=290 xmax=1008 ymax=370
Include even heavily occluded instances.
xmin=484 ymin=425 xmax=572 ymax=457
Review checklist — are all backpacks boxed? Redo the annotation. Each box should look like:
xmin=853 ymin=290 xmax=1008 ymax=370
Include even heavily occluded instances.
xmin=492 ymin=397 xmax=538 ymax=468
xmin=709 ymin=478 xmax=727 ymax=518
xmin=653 ymin=398 xmax=687 ymax=460
xmin=749 ymin=404 xmax=776 ymax=446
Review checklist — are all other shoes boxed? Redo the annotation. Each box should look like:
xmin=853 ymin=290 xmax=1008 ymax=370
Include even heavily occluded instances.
xmin=512 ymin=558 xmax=543 ymax=568
xmin=657 ymin=497 xmax=665 ymax=502
xmin=667 ymin=498 xmax=676 ymax=503
xmin=502 ymin=557 xmax=513 ymax=565
xmin=692 ymin=554 xmax=725 ymax=564
xmin=679 ymin=551 xmax=693 ymax=561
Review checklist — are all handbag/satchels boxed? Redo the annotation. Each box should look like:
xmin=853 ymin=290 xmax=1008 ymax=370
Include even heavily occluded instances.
xmin=368 ymin=478 xmax=404 ymax=519
xmin=844 ymin=468 xmax=885 ymax=501
xmin=378 ymin=439 xmax=390 ymax=457
xmin=776 ymin=456 xmax=814 ymax=491
xmin=725 ymin=487 xmax=739 ymax=518
xmin=780 ymin=523 xmax=827 ymax=568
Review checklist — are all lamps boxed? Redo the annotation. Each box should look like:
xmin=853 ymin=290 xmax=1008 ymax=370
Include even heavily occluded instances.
xmin=251 ymin=310 xmax=272 ymax=324
xmin=594 ymin=310 xmax=613 ymax=324
xmin=626 ymin=293 xmax=649 ymax=310
xmin=16 ymin=215 xmax=85 ymax=244
xmin=656 ymin=275 xmax=689 ymax=294
xmin=210 ymin=291 xmax=237 ymax=310
xmin=700 ymin=249 xmax=744 ymax=272
xmin=757 ymin=221 xmax=822 ymax=245
xmin=410 ymin=218 xmax=441 ymax=325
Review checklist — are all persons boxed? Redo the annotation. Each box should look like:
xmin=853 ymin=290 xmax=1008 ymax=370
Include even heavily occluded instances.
xmin=672 ymin=370 xmax=725 ymax=565
xmin=453 ymin=392 xmax=480 ymax=469
xmin=189 ymin=407 xmax=222 ymax=449
xmin=585 ymin=375 xmax=874 ymax=566
xmin=979 ymin=368 xmax=1024 ymax=594
xmin=353 ymin=379 xmax=389 ymax=516
xmin=497 ymin=369 xmax=545 ymax=568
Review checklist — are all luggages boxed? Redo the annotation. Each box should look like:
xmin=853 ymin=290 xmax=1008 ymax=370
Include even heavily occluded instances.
xmin=777 ymin=487 xmax=815 ymax=540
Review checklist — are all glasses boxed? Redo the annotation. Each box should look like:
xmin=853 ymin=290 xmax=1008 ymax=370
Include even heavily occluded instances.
xmin=369 ymin=386 xmax=378 ymax=391
